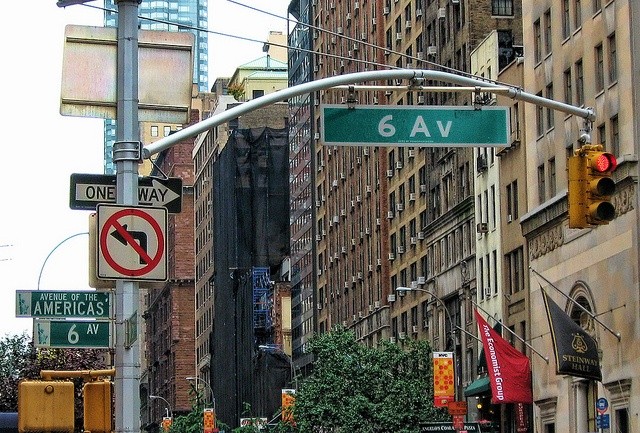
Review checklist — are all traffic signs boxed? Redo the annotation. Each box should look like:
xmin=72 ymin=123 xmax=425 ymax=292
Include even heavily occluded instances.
xmin=15 ymin=289 xmax=112 ymax=319
xmin=70 ymin=173 xmax=183 ymax=216
xmin=33 ymin=320 xmax=113 ymax=348
xmin=320 ymin=104 xmax=511 ymax=149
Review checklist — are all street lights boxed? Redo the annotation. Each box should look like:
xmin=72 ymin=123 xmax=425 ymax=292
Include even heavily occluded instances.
xmin=396 ymin=286 xmax=457 ymax=407
xmin=186 ymin=377 xmax=218 ymax=428
xmin=258 ymin=345 xmax=297 ymax=389
xmin=149 ymin=395 xmax=174 ymax=424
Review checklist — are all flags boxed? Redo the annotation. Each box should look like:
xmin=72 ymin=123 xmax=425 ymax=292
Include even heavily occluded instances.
xmin=538 ymin=283 xmax=603 ymax=382
xmin=473 ymin=308 xmax=532 ymax=405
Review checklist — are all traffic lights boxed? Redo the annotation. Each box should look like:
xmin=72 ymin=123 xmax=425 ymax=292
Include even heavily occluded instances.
xmin=567 ymin=144 xmax=615 ymax=228
